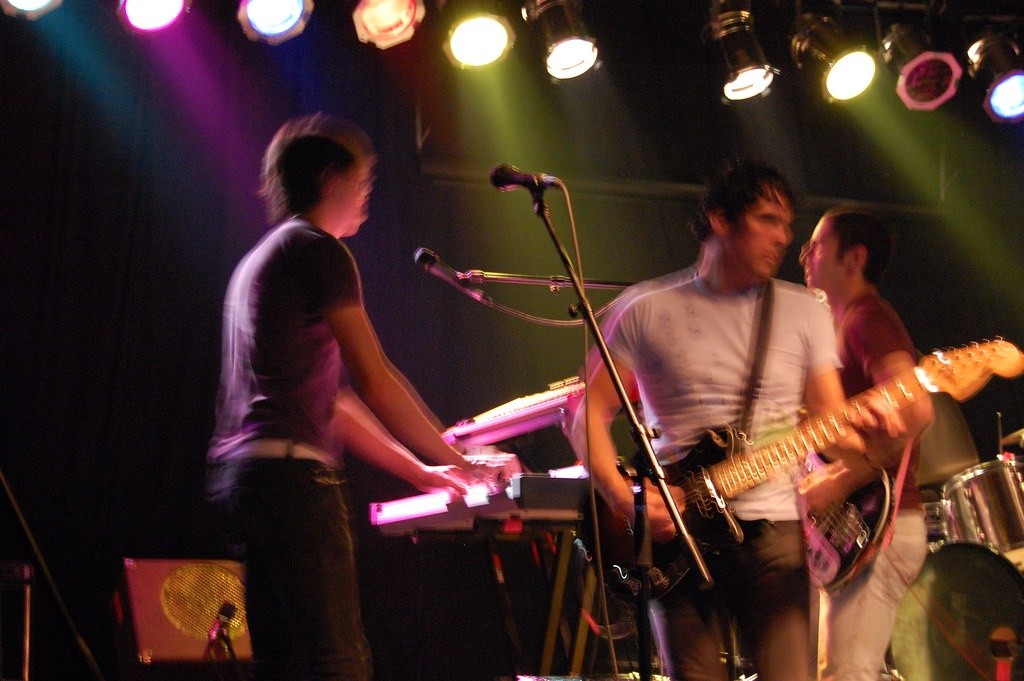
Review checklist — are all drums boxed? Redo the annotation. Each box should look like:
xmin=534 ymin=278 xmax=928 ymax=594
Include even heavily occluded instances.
xmin=876 ymin=536 xmax=1024 ymax=681
xmin=936 ymin=453 xmax=1022 ymax=569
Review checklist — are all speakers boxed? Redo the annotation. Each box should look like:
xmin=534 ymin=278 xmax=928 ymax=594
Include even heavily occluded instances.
xmin=111 ymin=557 xmax=258 ymax=681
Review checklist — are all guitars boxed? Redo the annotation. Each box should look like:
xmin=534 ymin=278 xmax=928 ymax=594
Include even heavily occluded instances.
xmin=793 ymin=443 xmax=895 ymax=588
xmin=580 ymin=335 xmax=1024 ymax=614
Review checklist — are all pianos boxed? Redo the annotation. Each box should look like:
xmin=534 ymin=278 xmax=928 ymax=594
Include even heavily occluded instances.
xmin=371 ymin=461 xmax=593 ymax=681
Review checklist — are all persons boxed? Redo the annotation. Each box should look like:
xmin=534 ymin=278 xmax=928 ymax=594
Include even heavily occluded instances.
xmin=568 ymin=161 xmax=866 ymax=680
xmin=205 ymin=121 xmax=527 ymax=681
xmin=796 ymin=203 xmax=934 ymax=681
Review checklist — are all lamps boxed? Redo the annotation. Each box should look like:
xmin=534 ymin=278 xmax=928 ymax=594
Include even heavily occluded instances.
xmin=873 ymin=2 xmax=962 ymax=112
xmin=779 ymin=0 xmax=880 ymax=105
xmin=698 ymin=0 xmax=780 ymax=106
xmin=521 ymin=0 xmax=599 ymax=80
xmin=437 ymin=0 xmax=516 ymax=72
xmin=200 ymin=0 xmax=315 ymax=46
xmin=105 ymin=0 xmax=192 ymax=34
xmin=320 ymin=0 xmax=426 ymax=53
xmin=951 ymin=0 xmax=1024 ymax=124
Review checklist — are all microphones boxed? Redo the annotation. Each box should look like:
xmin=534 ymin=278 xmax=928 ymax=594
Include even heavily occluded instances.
xmin=490 ymin=163 xmax=565 ymax=192
xmin=208 ymin=602 xmax=235 ymax=645
xmin=414 ymin=248 xmax=494 ymax=307
xmin=990 ymin=639 xmax=1024 ymax=658
